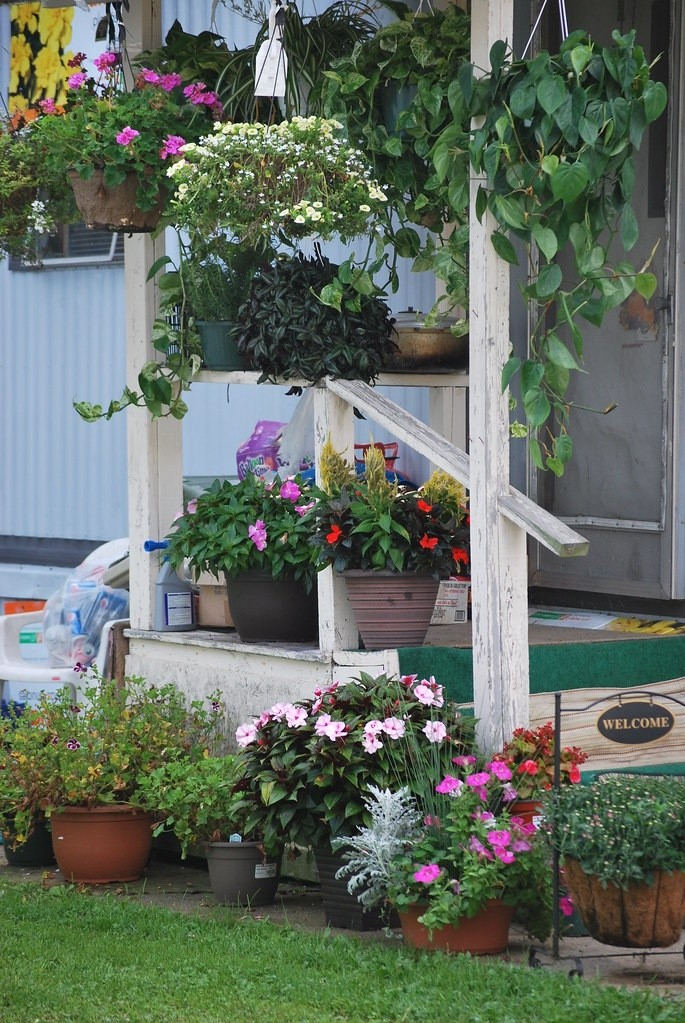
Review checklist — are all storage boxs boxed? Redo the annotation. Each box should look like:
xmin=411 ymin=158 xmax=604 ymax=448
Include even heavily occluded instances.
xmin=430 ymin=580 xmax=468 ymax=624
xmin=191 ymin=565 xmax=235 ymax=628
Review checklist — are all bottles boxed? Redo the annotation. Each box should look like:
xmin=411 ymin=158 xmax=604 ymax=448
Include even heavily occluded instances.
xmin=143 ymin=539 xmax=197 ymax=632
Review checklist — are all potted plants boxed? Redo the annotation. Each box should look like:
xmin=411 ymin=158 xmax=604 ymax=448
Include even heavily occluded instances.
xmin=183 ymin=219 xmax=298 ymax=372
xmin=129 ymin=747 xmax=283 ymax=906
xmin=214 ymin=0 xmax=383 ymax=123
xmin=308 ymin=0 xmax=469 ymax=339
xmin=419 ymin=27 xmax=670 ymax=479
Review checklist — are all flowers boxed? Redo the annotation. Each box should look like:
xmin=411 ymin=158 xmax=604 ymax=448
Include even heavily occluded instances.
xmin=166 ymin=114 xmax=390 ymax=257
xmin=330 ymin=673 xmax=591 ymax=944
xmin=0 ymin=663 xmax=228 ymax=854
xmin=534 ymin=772 xmax=685 ymax=892
xmin=228 ymin=670 xmax=481 ymax=856
xmin=155 ymin=467 xmax=316 ymax=585
xmin=30 ymin=49 xmax=226 ymax=211
xmin=295 ymin=429 xmax=471 ymax=584
xmin=488 ymin=719 xmax=589 ymax=800
xmin=0 ymin=110 xmax=69 ymax=274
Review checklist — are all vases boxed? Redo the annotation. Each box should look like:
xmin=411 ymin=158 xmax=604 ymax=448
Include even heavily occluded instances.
xmin=3 ymin=811 xmax=58 ymax=866
xmin=0 ymin=187 xmax=35 ymax=235
xmin=334 ymin=568 xmax=441 ymax=651
xmin=223 ymin=569 xmax=318 ymax=643
xmin=562 ymin=855 xmax=685 ymax=949
xmin=501 ymin=798 xmax=545 ymax=830
xmin=391 ymin=894 xmax=512 ymax=956
xmin=66 ymin=163 xmax=167 ymax=234
xmin=50 ymin=803 xmax=156 ymax=884
xmin=312 ymin=841 xmax=399 ymax=932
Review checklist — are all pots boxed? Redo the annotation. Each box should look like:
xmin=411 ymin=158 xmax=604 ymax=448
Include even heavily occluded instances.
xmin=377 ymin=307 xmax=469 ymax=370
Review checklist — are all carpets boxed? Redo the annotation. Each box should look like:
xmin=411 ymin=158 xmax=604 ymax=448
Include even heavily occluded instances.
xmin=396 ymin=635 xmax=685 ymax=938
